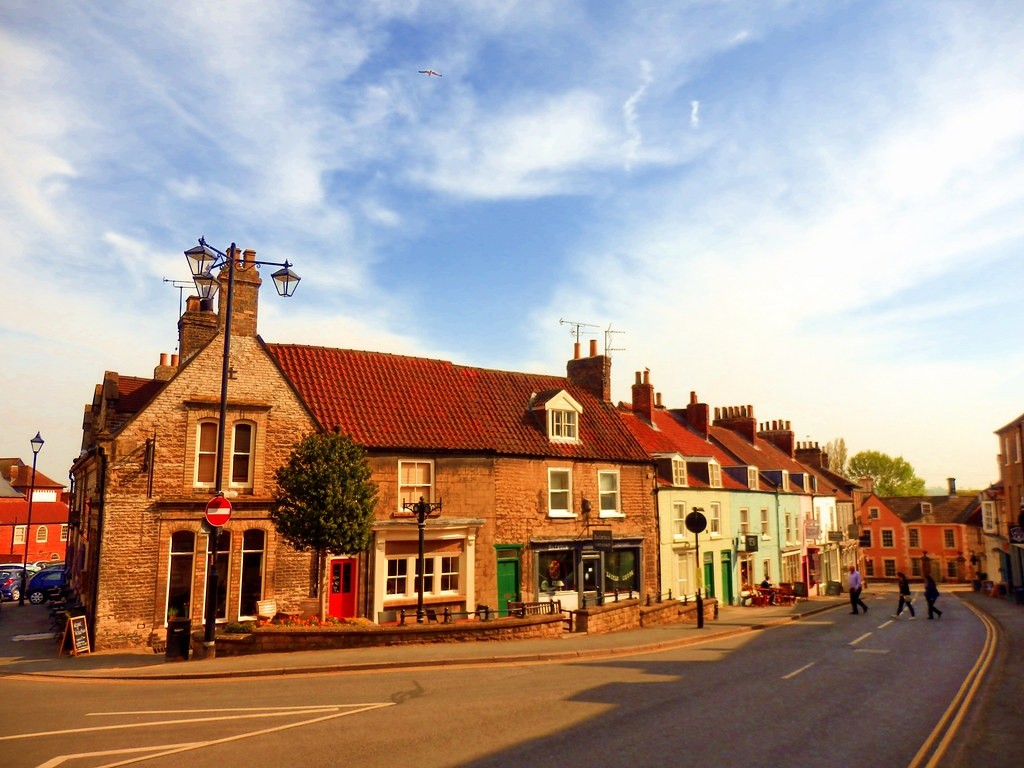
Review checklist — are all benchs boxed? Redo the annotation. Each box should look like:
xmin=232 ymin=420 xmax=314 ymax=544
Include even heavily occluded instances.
xmin=508 ymin=599 xmax=573 ymax=632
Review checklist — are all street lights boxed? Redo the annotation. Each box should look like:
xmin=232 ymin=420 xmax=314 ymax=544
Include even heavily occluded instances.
xmin=19 ymin=431 xmax=45 ymax=606
xmin=182 ymin=234 xmax=302 ymax=660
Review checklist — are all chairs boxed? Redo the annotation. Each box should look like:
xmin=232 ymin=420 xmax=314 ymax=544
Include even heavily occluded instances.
xmin=48 ymin=588 xmax=86 ymax=643
xmin=755 ymin=582 xmax=797 ymax=607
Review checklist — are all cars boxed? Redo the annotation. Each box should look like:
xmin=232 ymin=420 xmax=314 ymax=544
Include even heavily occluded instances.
xmin=0 ymin=561 xmax=65 ymax=605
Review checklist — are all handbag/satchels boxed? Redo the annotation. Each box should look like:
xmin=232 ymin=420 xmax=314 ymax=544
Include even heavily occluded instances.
xmin=903 ymin=595 xmax=913 ymax=602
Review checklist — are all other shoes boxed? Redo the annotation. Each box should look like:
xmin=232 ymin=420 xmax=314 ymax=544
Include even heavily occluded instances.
xmin=927 ymin=617 xmax=934 ymax=619
xmin=769 ymin=602 xmax=776 ymax=605
xmin=891 ymin=615 xmax=897 ymax=618
xmin=850 ymin=612 xmax=858 ymax=615
xmin=938 ymin=612 xmax=942 ymax=617
xmin=909 ymin=617 xmax=915 ymax=620
xmin=864 ymin=607 xmax=867 ymax=613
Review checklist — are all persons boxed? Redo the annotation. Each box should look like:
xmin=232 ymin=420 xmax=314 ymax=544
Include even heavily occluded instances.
xmin=923 ymin=574 xmax=943 ymax=620
xmin=848 ymin=566 xmax=869 ymax=614
xmin=890 ymin=572 xmax=916 ymax=620
xmin=760 ymin=575 xmax=776 ymax=605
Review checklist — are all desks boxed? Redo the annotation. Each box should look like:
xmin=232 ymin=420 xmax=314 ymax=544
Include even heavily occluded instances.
xmin=49 ymin=589 xmax=59 ymax=590
xmin=52 ymin=600 xmax=64 ymax=604
xmin=56 ymin=610 xmax=68 ymax=614
xmin=51 ymin=593 xmax=60 ymax=596
xmin=756 ymin=587 xmax=773 ymax=607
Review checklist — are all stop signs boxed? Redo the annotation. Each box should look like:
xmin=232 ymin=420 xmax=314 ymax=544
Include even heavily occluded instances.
xmin=205 ymin=496 xmax=232 ymax=527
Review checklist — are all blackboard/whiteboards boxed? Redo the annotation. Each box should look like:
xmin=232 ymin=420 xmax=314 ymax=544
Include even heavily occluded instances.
xmin=60 ymin=615 xmax=90 ymax=653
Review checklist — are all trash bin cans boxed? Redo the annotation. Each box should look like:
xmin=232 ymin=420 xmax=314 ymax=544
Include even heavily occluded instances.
xmin=972 ymin=580 xmax=981 ymax=591
xmin=166 ymin=618 xmax=191 ymax=661
